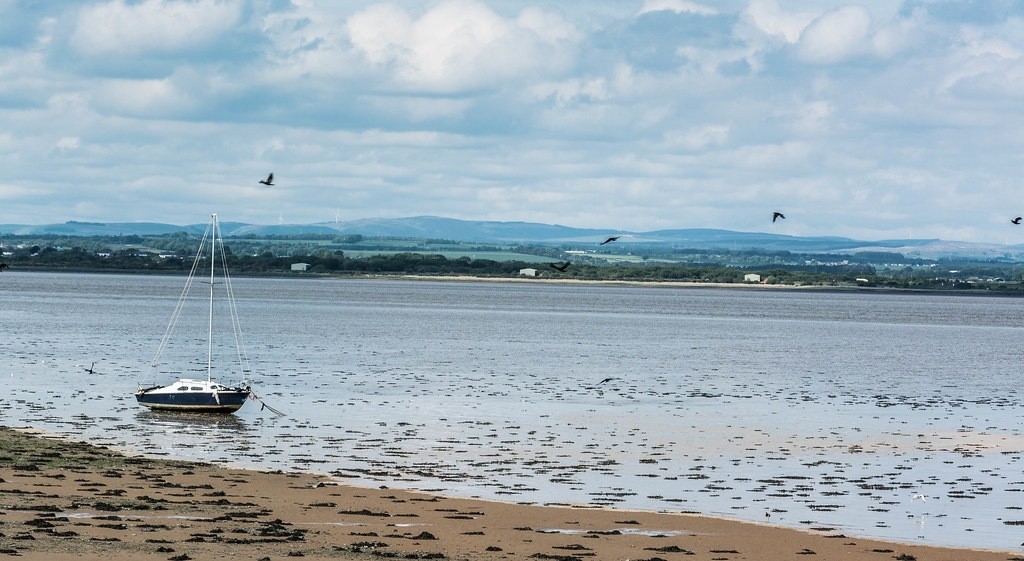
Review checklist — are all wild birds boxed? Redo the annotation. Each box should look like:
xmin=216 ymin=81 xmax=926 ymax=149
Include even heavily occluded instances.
xmin=599 ymin=235 xmax=621 ymax=246
xmin=596 ymin=377 xmax=623 ymax=385
xmin=1010 ymin=217 xmax=1022 ymax=225
xmin=84 ymin=362 xmax=95 ymax=375
xmin=549 ymin=262 xmax=571 ymax=273
xmin=772 ymin=211 xmax=788 ymax=223
xmin=258 ymin=172 xmax=276 ymax=187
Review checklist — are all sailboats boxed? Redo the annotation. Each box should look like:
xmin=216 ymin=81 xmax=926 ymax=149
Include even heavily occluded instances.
xmin=133 ymin=211 xmax=254 ymax=412
